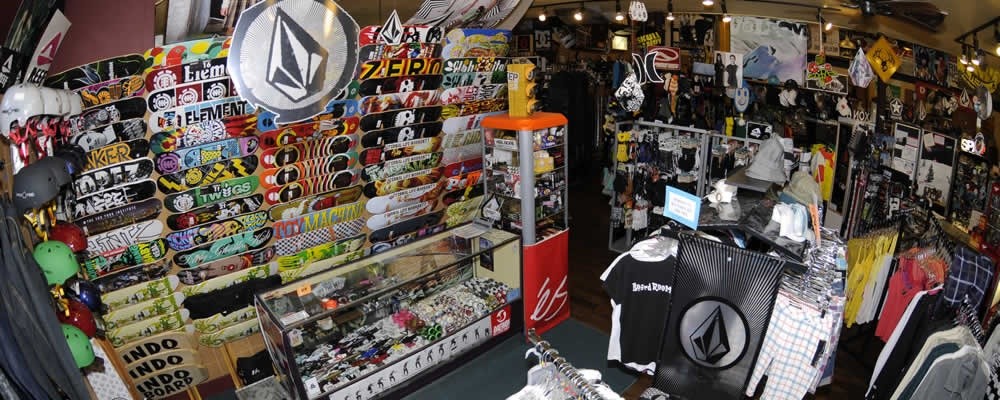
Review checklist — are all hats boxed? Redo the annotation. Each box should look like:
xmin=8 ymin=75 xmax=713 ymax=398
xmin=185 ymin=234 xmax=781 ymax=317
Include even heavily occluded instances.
xmin=744 ymin=136 xmax=787 ymax=184
xmin=783 ymin=172 xmax=823 ymax=208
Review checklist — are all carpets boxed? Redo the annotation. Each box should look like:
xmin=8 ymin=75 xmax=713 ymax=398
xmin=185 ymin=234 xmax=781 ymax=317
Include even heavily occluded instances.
xmin=403 ymin=317 xmax=641 ymax=400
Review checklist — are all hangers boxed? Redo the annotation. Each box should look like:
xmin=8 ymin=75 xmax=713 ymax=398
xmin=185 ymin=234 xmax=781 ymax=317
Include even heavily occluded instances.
xmin=779 ymin=224 xmax=839 ymax=312
xmin=524 ymin=341 xmax=603 ymax=400
xmin=932 ymin=294 xmax=986 ymax=369
xmin=627 ymin=221 xmax=677 ymax=261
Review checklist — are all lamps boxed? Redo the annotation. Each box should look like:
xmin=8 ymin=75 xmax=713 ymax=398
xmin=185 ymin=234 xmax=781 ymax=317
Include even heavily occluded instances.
xmin=531 ymin=0 xmax=840 ymax=31
xmin=954 ymin=16 xmax=1000 ymax=72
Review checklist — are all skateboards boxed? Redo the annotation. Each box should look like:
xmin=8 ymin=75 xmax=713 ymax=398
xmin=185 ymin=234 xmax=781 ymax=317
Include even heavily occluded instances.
xmin=358 ymin=23 xmax=448 ymax=255
xmin=144 ymin=36 xmax=285 ymax=349
xmin=41 ymin=53 xmax=209 ymax=400
xmin=256 ymin=60 xmax=371 ymax=286
xmin=442 ymin=27 xmax=518 ymax=229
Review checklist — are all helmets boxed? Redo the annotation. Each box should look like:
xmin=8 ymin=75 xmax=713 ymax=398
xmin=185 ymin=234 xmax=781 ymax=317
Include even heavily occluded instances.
xmin=68 ymin=279 xmax=102 ymax=312
xmin=61 ymin=324 xmax=95 ymax=369
xmin=0 ymin=83 xmax=44 ymax=139
xmin=56 ymin=298 xmax=96 ymax=339
xmin=57 ymin=150 xmax=83 ymax=175
xmin=63 ymin=92 xmax=85 ymax=122
xmin=49 ymin=223 xmax=88 ymax=253
xmin=38 ymin=157 xmax=72 ymax=187
xmin=53 ymin=90 xmax=71 ymax=123
xmin=33 ymin=240 xmax=81 ymax=285
xmin=64 ymin=144 xmax=86 ymax=163
xmin=14 ymin=164 xmax=60 ymax=213
xmin=36 ymin=87 xmax=61 ymax=130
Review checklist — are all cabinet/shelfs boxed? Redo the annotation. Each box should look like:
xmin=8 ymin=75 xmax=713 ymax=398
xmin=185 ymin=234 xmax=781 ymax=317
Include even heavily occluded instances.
xmin=479 ymin=111 xmax=570 ymax=338
